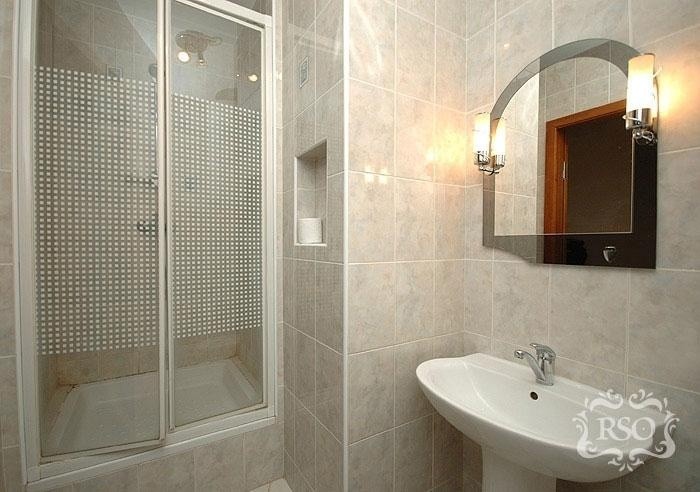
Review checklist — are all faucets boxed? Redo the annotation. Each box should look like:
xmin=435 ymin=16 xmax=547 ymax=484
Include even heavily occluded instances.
xmin=514 ymin=344 xmax=556 ymax=386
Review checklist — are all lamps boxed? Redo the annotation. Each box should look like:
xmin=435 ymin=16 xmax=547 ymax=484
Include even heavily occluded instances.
xmin=474 ymin=111 xmax=494 ymax=177
xmin=622 ymin=52 xmax=659 ymax=148
xmin=492 ymin=117 xmax=506 ymax=175
xmin=175 ymin=29 xmax=222 ymax=69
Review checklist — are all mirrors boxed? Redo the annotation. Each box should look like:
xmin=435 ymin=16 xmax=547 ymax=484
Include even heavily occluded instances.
xmin=482 ymin=38 xmax=656 ymax=269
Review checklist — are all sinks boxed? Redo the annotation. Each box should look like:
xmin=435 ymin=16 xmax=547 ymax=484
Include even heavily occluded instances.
xmin=416 ymin=354 xmax=677 ymax=484
